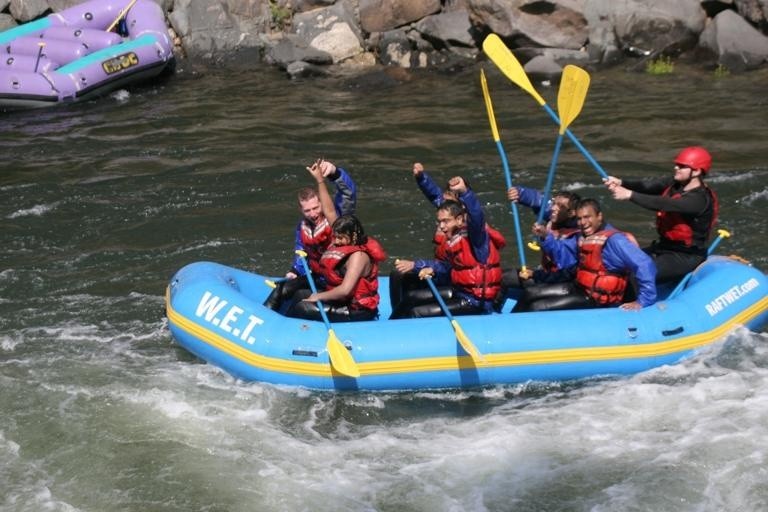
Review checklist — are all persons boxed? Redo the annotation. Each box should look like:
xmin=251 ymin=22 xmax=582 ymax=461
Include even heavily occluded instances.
xmin=507 ymin=185 xmax=603 ymax=284
xmin=263 ymin=158 xmax=357 ymax=313
xmin=602 ymin=146 xmax=718 ymax=300
xmin=390 ymin=175 xmax=507 ymax=319
xmin=510 ymin=197 xmax=658 ymax=313
xmin=281 ymin=158 xmax=387 ymax=322
xmin=388 ymin=163 xmax=506 ymax=309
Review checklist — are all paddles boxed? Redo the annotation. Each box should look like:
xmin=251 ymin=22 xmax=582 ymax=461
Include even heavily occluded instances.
xmin=478 ymin=33 xmax=606 ymax=279
xmin=294 ymin=248 xmax=362 ymax=378
xmin=424 ymin=273 xmax=478 ymax=356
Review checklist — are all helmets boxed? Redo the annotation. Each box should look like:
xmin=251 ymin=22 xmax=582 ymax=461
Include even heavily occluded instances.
xmin=671 ymin=145 xmax=712 ymax=173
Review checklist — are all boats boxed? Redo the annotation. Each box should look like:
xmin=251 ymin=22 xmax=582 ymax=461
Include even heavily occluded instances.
xmin=0 ymin=0 xmax=172 ymax=108
xmin=164 ymin=252 xmax=768 ymax=394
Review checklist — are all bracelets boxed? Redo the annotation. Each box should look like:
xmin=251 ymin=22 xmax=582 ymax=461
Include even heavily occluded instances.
xmin=317 ymin=178 xmax=325 ymax=185
